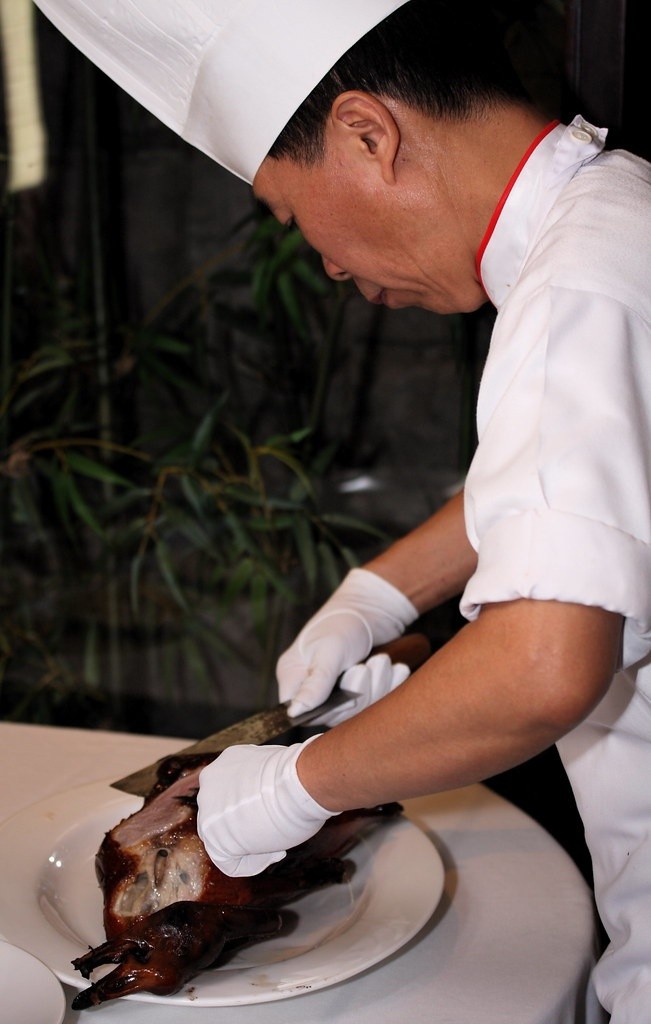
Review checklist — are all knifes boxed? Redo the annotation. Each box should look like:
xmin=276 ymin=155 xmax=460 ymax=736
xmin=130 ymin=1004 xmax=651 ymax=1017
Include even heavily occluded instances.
xmin=108 ymin=633 xmax=433 ymax=798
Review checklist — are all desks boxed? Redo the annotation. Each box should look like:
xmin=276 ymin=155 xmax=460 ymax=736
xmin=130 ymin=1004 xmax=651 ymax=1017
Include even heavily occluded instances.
xmin=0 ymin=719 xmax=605 ymax=1024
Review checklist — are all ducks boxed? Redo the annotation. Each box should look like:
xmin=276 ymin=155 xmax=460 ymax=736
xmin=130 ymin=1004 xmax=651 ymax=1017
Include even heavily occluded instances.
xmin=70 ymin=723 xmax=407 ymax=1013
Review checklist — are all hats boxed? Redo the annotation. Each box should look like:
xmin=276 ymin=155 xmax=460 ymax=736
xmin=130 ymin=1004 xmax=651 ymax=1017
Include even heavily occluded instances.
xmin=34 ymin=0 xmax=408 ymax=187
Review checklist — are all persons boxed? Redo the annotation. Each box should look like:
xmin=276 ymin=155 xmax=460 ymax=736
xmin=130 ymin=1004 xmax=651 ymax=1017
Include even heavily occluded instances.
xmin=40 ymin=0 xmax=651 ymax=1023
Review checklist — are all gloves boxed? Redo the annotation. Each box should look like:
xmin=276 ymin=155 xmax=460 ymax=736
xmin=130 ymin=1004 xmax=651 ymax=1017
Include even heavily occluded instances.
xmin=196 ymin=733 xmax=343 ymax=878
xmin=274 ymin=567 xmax=411 ymax=729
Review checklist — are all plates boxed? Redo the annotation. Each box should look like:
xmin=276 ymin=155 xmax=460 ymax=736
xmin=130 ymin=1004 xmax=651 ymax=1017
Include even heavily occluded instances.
xmin=0 ymin=940 xmax=67 ymax=1024
xmin=1 ymin=779 xmax=445 ymax=1008
